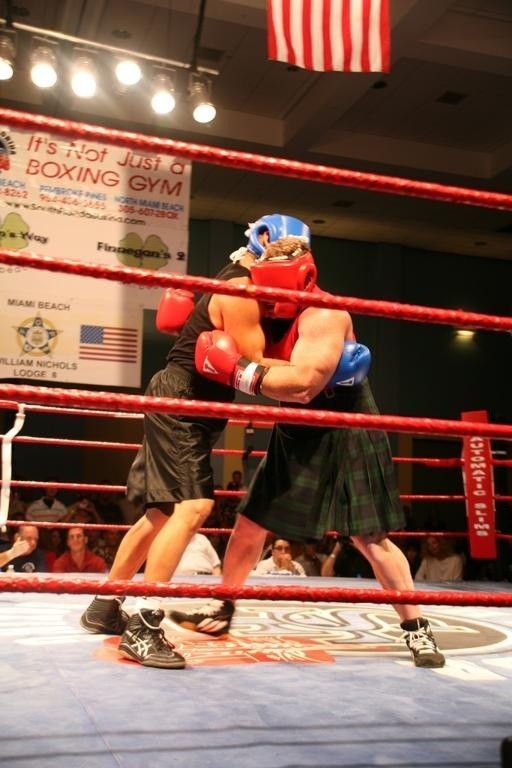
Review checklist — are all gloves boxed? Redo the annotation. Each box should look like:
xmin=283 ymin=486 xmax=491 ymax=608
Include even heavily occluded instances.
xmin=326 ymin=341 xmax=372 ymax=387
xmin=193 ymin=328 xmax=271 ymax=401
xmin=155 ymin=285 xmax=198 ymax=336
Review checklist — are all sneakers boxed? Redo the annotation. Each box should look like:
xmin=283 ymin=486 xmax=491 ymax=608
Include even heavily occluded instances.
xmin=400 ymin=617 xmax=446 ymax=669
xmin=80 ymin=593 xmax=132 ymax=635
xmin=166 ymin=599 xmax=235 ymax=640
xmin=119 ymin=607 xmax=189 ymax=668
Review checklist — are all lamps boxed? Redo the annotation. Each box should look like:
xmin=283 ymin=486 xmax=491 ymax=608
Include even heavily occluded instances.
xmin=0 ymin=0 xmax=221 ymax=127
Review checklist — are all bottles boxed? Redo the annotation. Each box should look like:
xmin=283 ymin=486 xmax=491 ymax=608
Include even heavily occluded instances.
xmin=7 ymin=565 xmax=15 ymax=574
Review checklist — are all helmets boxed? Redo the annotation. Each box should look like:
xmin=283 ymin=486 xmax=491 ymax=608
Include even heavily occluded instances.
xmin=249 ymin=253 xmax=318 ymax=319
xmin=244 ymin=212 xmax=314 ymax=260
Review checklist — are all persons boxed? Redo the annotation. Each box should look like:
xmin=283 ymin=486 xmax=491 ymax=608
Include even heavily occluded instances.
xmin=80 ymin=214 xmax=372 ymax=669
xmin=166 ymin=237 xmax=445 ymax=669
xmin=0 ymin=472 xmax=462 ymax=582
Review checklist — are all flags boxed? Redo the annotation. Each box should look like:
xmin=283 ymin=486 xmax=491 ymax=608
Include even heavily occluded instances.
xmin=79 ymin=325 xmax=138 ymax=362
xmin=266 ymin=0 xmax=391 ymax=73
